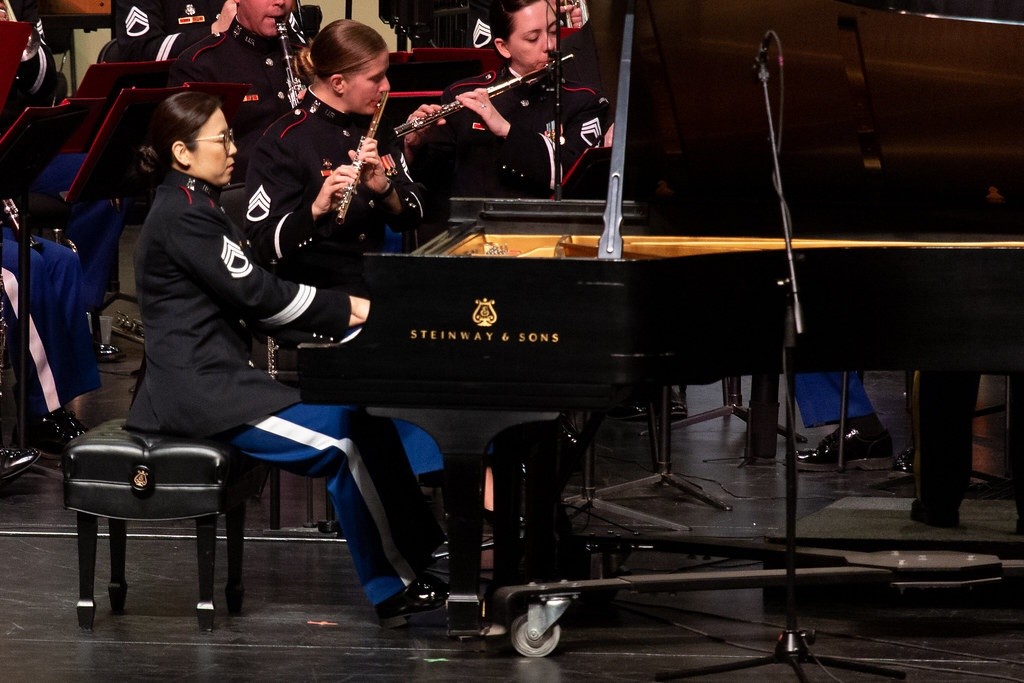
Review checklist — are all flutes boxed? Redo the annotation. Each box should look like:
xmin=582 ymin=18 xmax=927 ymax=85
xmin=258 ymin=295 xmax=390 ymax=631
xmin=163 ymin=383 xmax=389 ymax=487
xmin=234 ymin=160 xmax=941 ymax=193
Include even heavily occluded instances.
xmin=392 ymin=53 xmax=574 ymax=139
xmin=275 ymin=16 xmax=303 ymax=109
xmin=336 ymin=91 xmax=389 ymax=225
xmin=3 ymin=199 xmax=44 ymax=251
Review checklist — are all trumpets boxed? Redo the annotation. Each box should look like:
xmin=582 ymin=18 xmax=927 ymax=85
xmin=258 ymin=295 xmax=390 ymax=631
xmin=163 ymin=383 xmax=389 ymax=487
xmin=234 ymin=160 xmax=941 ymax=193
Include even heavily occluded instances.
xmin=0 ymin=0 xmax=42 ymax=62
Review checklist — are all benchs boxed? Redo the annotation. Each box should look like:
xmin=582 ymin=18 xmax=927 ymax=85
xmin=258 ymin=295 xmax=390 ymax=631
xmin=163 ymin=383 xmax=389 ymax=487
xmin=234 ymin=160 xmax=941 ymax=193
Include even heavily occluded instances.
xmin=62 ymin=418 xmax=260 ymax=634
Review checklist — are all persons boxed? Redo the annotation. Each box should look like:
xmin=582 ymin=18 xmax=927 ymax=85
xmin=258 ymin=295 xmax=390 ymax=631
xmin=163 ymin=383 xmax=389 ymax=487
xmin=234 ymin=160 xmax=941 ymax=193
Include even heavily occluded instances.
xmin=115 ymin=0 xmax=313 ymax=186
xmin=911 ymin=373 xmax=1024 ymax=533
xmin=793 ymin=368 xmax=914 ymax=472
xmin=245 ymin=21 xmax=497 ymax=573
xmin=402 ymin=1 xmax=610 ymax=559
xmin=0 ymin=0 xmax=126 ymax=480
xmin=120 ymin=92 xmax=495 ymax=632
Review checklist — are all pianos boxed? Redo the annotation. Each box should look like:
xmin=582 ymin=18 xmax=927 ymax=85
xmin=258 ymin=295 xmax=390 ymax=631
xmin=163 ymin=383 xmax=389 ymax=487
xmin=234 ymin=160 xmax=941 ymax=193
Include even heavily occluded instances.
xmin=293 ymin=0 xmax=1024 ymax=642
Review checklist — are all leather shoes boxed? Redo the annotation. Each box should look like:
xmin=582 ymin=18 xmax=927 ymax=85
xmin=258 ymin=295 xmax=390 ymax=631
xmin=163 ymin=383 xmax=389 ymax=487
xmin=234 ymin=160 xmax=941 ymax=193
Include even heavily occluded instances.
xmin=896 ymin=446 xmax=914 ymax=473
xmin=795 ymin=425 xmax=895 ymax=471
xmin=0 ymin=447 xmax=42 ymax=480
xmin=10 ymin=410 xmax=90 ymax=459
xmin=376 ymin=570 xmax=450 ymax=627
xmin=96 ymin=342 xmax=126 ymax=363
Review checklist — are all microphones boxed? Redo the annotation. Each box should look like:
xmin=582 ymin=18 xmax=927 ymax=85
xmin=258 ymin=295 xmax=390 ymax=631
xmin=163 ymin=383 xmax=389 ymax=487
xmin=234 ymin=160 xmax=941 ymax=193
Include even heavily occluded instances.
xmin=753 ymin=31 xmax=773 ymax=77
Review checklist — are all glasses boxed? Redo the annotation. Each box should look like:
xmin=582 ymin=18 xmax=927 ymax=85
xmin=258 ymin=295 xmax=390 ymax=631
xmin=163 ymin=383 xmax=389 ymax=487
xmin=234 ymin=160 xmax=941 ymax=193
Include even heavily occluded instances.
xmin=183 ymin=128 xmax=234 ymax=152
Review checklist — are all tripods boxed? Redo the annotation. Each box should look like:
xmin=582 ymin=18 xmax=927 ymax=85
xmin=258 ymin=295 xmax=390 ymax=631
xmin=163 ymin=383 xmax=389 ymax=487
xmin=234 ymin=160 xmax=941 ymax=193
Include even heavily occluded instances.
xmin=657 ymin=63 xmax=907 ymax=683
